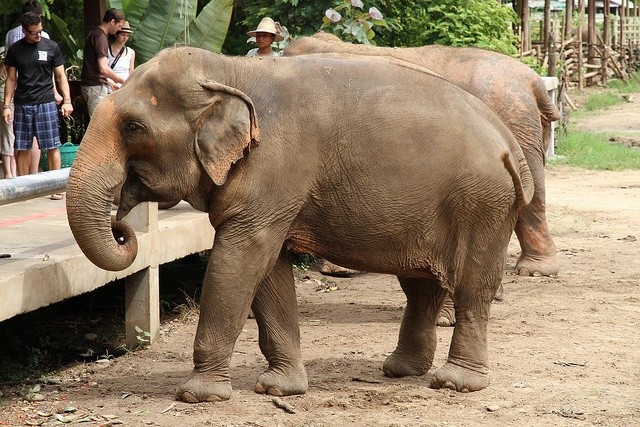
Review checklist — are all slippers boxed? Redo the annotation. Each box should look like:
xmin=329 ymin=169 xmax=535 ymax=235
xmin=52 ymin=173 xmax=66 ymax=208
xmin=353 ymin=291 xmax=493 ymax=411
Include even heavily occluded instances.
xmin=50 ymin=193 xmax=63 ymax=199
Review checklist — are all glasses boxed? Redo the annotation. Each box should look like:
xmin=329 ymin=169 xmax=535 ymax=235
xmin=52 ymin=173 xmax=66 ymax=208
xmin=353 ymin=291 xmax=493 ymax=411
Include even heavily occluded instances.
xmin=27 ymin=30 xmax=42 ymax=35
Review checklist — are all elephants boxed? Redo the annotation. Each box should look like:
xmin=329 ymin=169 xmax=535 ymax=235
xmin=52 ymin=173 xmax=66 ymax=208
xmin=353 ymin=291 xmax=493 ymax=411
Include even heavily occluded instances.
xmin=283 ymin=30 xmax=564 ymax=278
xmin=65 ymin=45 xmax=537 ymax=403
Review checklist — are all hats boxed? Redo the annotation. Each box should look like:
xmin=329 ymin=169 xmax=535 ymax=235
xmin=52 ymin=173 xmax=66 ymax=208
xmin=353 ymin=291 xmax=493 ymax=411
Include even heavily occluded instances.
xmin=119 ymin=20 xmax=133 ymax=32
xmin=246 ymin=17 xmax=283 ymax=41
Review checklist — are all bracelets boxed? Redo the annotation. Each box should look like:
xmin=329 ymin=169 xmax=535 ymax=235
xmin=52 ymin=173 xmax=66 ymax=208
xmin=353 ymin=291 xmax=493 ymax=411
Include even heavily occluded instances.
xmin=2 ymin=103 xmax=14 ymax=111
xmin=64 ymin=98 xmax=72 ymax=104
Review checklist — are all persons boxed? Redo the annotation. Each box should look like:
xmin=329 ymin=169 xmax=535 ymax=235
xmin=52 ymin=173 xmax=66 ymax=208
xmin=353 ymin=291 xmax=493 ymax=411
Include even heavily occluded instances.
xmin=107 ymin=20 xmax=136 ymax=94
xmin=80 ymin=8 xmax=126 ymax=125
xmin=244 ymin=17 xmax=285 ymax=58
xmin=0 ymin=41 xmax=17 ymax=180
xmin=5 ymin=1 xmax=63 ymax=179
xmin=2 ymin=13 xmax=74 ymax=200
